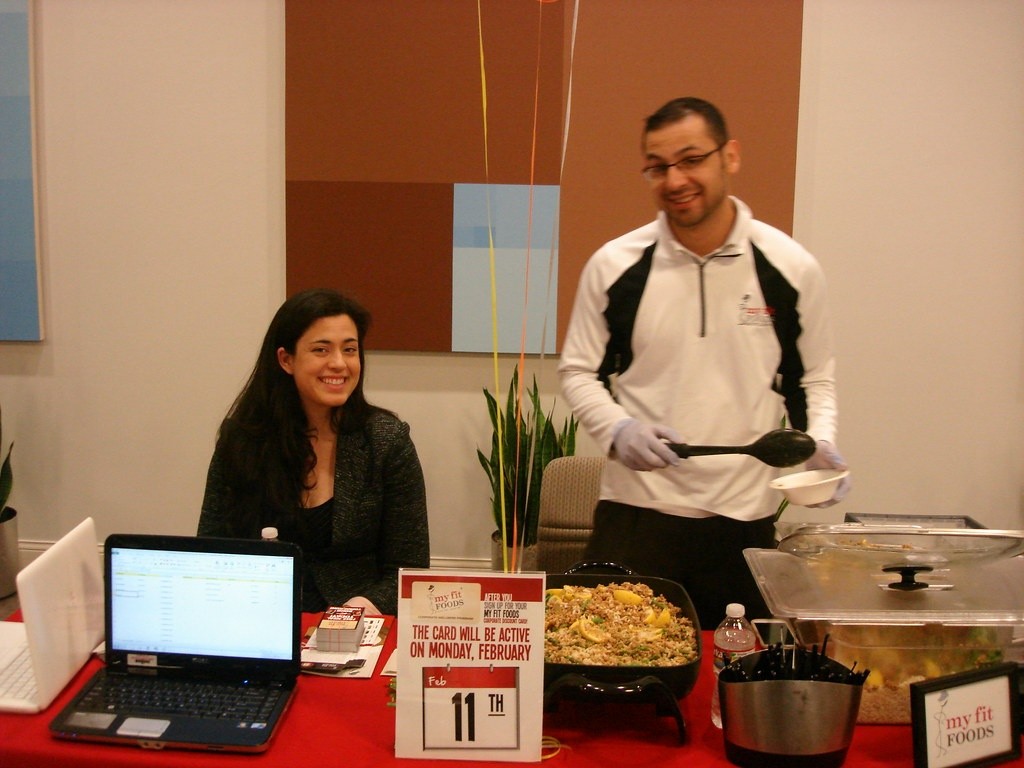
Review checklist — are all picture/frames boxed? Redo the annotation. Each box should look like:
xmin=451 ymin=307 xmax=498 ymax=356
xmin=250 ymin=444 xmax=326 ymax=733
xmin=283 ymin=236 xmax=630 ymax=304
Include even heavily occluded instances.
xmin=910 ymin=661 xmax=1024 ymax=768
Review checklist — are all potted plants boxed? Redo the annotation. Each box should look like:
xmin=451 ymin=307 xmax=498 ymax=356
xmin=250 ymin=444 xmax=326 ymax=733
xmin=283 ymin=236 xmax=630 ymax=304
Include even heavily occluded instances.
xmin=0 ymin=406 xmax=19 ymax=599
xmin=475 ymin=363 xmax=580 ymax=573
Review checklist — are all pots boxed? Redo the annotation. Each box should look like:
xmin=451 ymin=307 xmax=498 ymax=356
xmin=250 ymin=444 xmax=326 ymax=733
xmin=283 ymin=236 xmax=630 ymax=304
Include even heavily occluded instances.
xmin=544 ymin=559 xmax=702 ymax=746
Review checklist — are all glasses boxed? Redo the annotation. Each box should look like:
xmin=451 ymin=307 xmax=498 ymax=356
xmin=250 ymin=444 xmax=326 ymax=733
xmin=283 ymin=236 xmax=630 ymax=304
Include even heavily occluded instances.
xmin=640 ymin=142 xmax=728 ymax=180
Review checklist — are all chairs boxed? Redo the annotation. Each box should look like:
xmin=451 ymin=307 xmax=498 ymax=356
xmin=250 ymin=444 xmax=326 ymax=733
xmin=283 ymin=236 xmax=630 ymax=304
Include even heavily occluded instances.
xmin=536 ymin=456 xmax=607 ymax=590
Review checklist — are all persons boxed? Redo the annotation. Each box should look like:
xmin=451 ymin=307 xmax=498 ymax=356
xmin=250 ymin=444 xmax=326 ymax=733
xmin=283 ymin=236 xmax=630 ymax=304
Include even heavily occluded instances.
xmin=557 ymin=96 xmax=851 ymax=639
xmin=196 ymin=288 xmax=430 ymax=616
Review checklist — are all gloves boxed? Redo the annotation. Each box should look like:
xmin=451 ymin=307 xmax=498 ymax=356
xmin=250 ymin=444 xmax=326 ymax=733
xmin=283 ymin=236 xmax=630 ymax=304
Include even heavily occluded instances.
xmin=806 ymin=441 xmax=849 ymax=509
xmin=613 ymin=418 xmax=687 ymax=470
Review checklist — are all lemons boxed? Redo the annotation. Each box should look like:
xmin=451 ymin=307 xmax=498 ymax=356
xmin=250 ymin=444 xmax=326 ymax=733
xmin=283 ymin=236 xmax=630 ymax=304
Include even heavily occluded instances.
xmin=864 ymin=646 xmax=942 ymax=688
xmin=544 ymin=586 xmax=671 ymax=644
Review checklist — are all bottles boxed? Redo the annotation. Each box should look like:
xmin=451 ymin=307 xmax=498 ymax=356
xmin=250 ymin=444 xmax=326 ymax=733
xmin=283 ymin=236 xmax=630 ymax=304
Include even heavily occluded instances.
xmin=711 ymin=604 xmax=757 ymax=729
xmin=261 ymin=527 xmax=279 ymax=541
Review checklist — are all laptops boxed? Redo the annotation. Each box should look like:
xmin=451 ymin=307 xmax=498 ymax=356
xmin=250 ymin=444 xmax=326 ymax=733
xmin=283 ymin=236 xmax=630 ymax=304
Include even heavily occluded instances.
xmin=49 ymin=534 xmax=304 ymax=753
xmin=0 ymin=516 xmax=108 ymax=714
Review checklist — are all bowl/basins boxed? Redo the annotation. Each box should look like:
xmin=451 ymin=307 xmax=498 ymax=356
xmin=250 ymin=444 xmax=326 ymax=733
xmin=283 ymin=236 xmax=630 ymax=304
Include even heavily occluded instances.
xmin=718 ymin=648 xmax=864 ymax=768
xmin=770 ymin=468 xmax=850 ymax=506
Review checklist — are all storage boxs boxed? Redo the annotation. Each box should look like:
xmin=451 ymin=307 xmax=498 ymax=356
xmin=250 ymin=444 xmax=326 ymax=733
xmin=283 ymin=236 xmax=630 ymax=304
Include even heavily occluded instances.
xmin=803 ymin=552 xmax=1024 ymax=724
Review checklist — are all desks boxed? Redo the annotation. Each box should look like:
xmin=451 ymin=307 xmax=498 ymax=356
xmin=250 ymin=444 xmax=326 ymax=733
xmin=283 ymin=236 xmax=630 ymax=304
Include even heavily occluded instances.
xmin=0 ymin=605 xmax=1024 ymax=768
